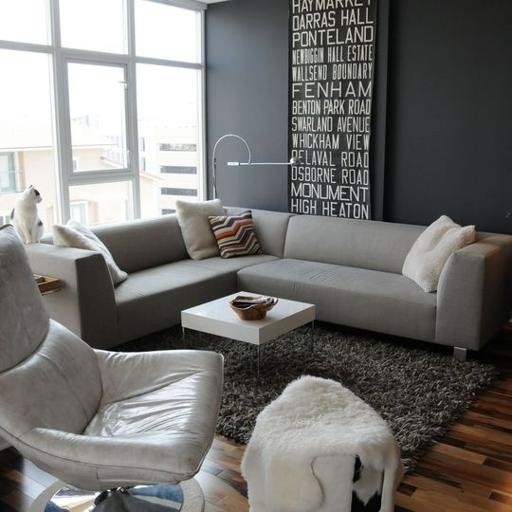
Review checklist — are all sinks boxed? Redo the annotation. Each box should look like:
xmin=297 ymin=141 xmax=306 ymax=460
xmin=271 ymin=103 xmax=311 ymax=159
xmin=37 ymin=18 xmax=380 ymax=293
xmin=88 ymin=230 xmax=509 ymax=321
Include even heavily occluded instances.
xmin=22 ymin=206 xmax=512 ymax=363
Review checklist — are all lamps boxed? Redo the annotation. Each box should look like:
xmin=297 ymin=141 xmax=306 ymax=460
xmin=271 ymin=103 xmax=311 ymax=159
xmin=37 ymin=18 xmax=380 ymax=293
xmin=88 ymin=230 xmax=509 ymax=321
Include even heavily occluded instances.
xmin=0 ymin=221 xmax=229 ymax=511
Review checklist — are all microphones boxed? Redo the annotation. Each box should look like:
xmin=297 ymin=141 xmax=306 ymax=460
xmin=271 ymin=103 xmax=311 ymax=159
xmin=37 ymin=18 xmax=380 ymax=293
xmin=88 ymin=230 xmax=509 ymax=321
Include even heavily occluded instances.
xmin=351 ymin=450 xmax=387 ymax=511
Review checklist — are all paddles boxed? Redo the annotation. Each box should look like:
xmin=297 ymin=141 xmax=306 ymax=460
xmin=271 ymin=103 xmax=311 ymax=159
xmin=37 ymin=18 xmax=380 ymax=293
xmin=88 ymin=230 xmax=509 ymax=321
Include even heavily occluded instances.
xmin=105 ymin=319 xmax=501 ymax=476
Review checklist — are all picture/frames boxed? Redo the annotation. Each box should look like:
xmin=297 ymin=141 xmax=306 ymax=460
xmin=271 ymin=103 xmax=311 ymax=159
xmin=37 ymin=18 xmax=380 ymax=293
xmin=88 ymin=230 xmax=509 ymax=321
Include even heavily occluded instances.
xmin=34 ymin=273 xmax=64 ymax=296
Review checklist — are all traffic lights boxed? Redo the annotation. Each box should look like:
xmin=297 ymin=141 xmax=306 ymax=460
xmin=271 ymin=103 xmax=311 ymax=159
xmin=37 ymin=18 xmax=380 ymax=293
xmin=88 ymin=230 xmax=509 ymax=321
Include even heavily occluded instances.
xmin=52 ymin=218 xmax=129 ymax=288
xmin=207 ymin=209 xmax=266 ymax=259
xmin=401 ymin=213 xmax=477 ymax=294
xmin=174 ymin=197 xmax=227 ymax=262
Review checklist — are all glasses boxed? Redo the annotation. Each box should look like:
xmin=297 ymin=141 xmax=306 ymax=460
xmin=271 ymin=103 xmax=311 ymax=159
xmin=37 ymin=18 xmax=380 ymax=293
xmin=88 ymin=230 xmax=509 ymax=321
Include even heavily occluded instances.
xmin=210 ymin=132 xmax=302 ymax=200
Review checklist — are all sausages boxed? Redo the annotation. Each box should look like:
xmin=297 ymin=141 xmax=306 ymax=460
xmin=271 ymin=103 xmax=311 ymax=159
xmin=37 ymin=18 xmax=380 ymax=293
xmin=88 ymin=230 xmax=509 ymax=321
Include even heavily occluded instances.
xmin=9 ymin=184 xmax=45 ymax=244
